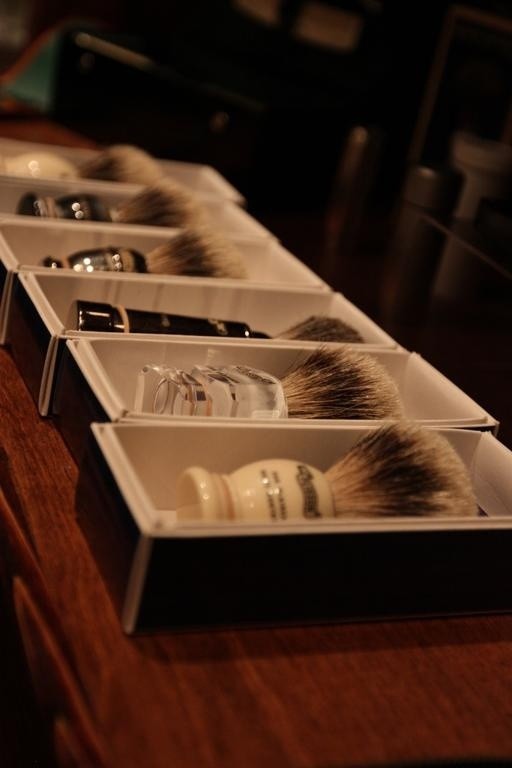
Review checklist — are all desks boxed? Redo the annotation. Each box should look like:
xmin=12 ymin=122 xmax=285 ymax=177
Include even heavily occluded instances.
xmin=1 ymin=96 xmax=510 ymax=767
